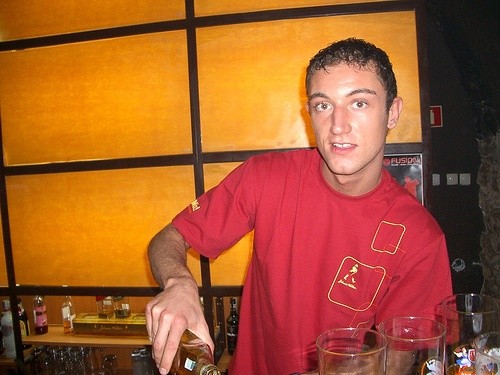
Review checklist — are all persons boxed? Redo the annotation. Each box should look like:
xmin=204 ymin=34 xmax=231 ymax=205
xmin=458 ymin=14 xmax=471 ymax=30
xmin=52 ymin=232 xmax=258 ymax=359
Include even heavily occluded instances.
xmin=145 ymin=37 xmax=460 ymax=375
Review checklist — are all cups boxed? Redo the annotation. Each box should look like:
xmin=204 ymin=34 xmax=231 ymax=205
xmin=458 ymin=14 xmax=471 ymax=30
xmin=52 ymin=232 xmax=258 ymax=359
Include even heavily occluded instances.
xmin=94 ymin=295 xmax=131 ymax=318
xmin=315 ymin=293 xmax=500 ymax=375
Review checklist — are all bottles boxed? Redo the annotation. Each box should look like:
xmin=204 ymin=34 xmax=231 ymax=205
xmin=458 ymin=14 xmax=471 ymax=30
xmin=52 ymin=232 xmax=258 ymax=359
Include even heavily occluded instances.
xmin=171 ymin=330 xmax=220 ymax=375
xmin=62 ymin=296 xmax=76 ymax=336
xmin=30 ymin=345 xmax=150 ymax=375
xmin=227 ymin=297 xmax=239 ymax=355
xmin=33 ymin=295 xmax=48 ymax=334
xmin=0 ymin=284 xmax=32 ymax=358
